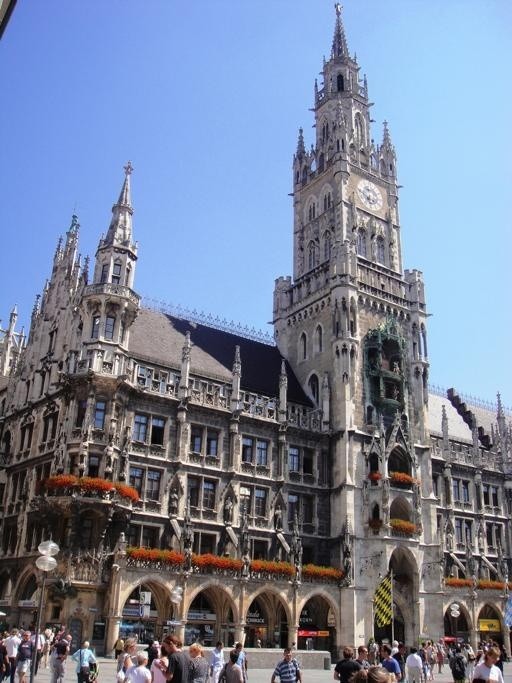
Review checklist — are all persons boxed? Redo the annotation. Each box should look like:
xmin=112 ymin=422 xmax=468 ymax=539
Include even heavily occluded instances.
xmin=334 ymin=638 xmax=507 ymax=683
xmin=0 ymin=625 xmax=97 ymax=683
xmin=272 ymin=642 xmax=302 ymax=682
xmin=256 ymin=639 xmax=261 ymax=648
xmin=114 ymin=635 xmax=247 ymax=683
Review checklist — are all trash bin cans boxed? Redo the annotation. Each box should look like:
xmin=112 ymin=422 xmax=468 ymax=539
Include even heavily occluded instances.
xmin=324 ymin=658 xmax=331 ymax=670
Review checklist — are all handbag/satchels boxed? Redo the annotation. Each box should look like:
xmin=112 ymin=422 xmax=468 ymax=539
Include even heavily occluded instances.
xmin=80 ymin=666 xmax=90 ymax=680
xmin=219 ymin=663 xmax=228 ymax=682
xmin=116 ymin=654 xmax=129 ymax=682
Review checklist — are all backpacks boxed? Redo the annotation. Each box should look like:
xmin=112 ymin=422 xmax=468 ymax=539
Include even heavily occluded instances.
xmin=450 ymin=655 xmax=465 ymax=682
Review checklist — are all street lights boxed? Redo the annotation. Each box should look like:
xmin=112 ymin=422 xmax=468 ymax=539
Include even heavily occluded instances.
xmin=447 ymin=603 xmax=462 ymax=638
xmin=29 ymin=531 xmax=62 ymax=682
xmin=167 ymin=585 xmax=184 ymax=638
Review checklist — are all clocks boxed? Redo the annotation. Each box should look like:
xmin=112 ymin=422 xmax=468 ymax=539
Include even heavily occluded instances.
xmin=355 ymin=179 xmax=383 ymax=211
